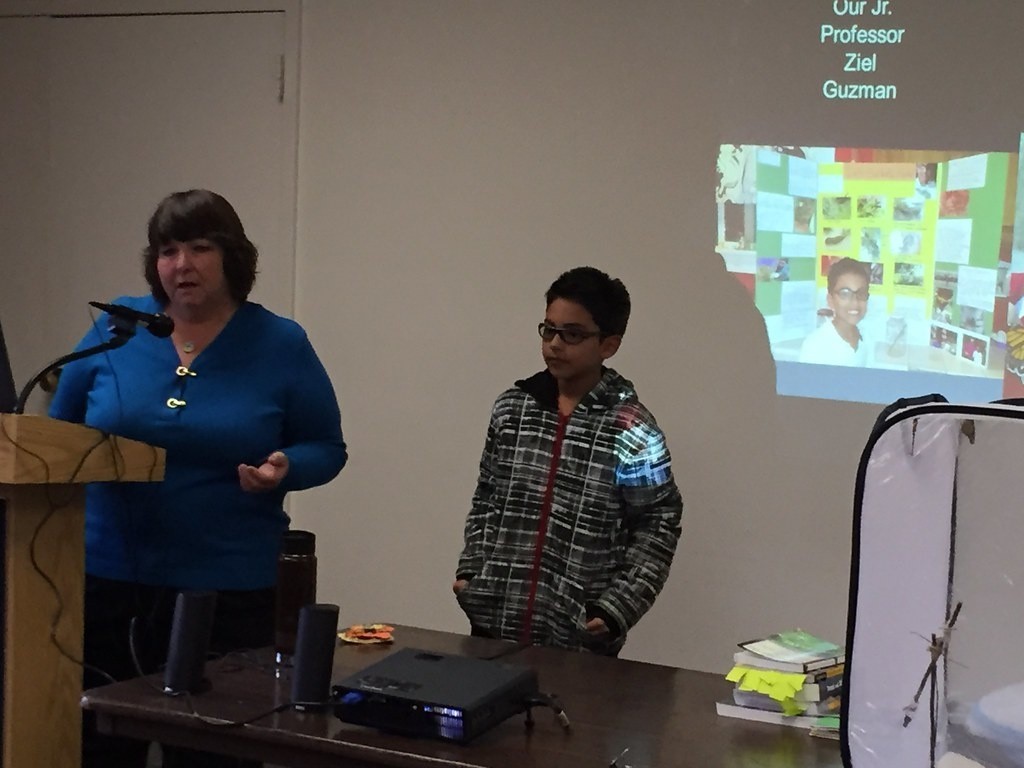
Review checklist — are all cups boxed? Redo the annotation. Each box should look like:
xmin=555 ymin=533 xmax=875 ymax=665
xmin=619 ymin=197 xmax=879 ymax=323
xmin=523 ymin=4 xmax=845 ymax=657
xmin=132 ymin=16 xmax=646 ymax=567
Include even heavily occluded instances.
xmin=289 ymin=602 xmax=340 ymax=712
xmin=275 ymin=529 xmax=318 ymax=667
xmin=163 ymin=591 xmax=217 ymax=697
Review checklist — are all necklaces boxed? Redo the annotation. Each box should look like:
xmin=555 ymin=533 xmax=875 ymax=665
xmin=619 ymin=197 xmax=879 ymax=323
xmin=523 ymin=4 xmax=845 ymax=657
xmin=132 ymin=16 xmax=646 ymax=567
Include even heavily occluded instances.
xmin=183 ymin=342 xmax=195 ymax=352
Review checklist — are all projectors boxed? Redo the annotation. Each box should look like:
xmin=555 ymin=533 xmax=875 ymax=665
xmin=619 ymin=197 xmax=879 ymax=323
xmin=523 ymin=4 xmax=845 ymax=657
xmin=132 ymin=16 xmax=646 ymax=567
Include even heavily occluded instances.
xmin=332 ymin=646 xmax=544 ymax=747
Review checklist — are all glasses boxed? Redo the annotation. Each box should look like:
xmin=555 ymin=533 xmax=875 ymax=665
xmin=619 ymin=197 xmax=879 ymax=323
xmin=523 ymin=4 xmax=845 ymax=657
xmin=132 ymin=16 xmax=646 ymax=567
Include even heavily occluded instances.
xmin=835 ymin=289 xmax=871 ymax=303
xmin=537 ymin=323 xmax=611 ymax=346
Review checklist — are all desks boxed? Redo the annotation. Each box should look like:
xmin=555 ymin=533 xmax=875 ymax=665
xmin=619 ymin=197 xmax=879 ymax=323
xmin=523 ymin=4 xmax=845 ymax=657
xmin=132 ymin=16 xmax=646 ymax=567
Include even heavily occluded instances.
xmin=76 ymin=623 xmax=1024 ymax=768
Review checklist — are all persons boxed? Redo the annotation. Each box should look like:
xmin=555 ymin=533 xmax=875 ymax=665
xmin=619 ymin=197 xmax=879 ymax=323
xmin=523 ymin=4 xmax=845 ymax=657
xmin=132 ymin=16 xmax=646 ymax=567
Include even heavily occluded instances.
xmin=801 ymin=257 xmax=870 ymax=365
xmin=454 ymin=268 xmax=683 ymax=657
xmin=48 ymin=189 xmax=347 ymax=767
xmin=916 ymin=163 xmax=935 ymax=189
xmin=770 ymin=258 xmax=791 ymax=281
xmin=963 ymin=339 xmax=985 ymax=364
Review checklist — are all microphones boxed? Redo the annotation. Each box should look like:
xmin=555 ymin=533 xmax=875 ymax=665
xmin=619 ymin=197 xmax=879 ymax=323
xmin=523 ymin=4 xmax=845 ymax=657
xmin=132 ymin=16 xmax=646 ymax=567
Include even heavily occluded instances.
xmin=87 ymin=301 xmax=175 ymax=337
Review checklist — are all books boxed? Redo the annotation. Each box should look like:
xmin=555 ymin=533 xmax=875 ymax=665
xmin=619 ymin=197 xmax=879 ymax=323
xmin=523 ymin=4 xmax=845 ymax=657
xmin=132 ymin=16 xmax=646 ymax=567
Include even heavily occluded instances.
xmin=715 ymin=630 xmax=844 ymax=731
